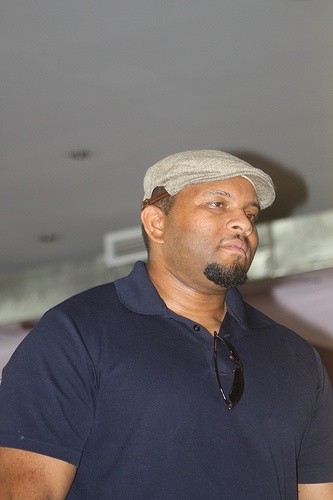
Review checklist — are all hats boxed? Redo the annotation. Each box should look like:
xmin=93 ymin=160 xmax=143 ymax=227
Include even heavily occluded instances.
xmin=143 ymin=149 xmax=276 ymax=211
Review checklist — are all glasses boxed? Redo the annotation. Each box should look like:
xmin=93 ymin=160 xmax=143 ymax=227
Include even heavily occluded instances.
xmin=212 ymin=331 xmax=245 ymax=410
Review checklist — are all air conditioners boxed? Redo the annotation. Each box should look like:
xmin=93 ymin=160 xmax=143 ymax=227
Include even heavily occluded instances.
xmin=104 ymin=227 xmax=147 ymax=268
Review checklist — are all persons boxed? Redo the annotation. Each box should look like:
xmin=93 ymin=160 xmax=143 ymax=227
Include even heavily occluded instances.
xmin=0 ymin=149 xmax=333 ymax=500
